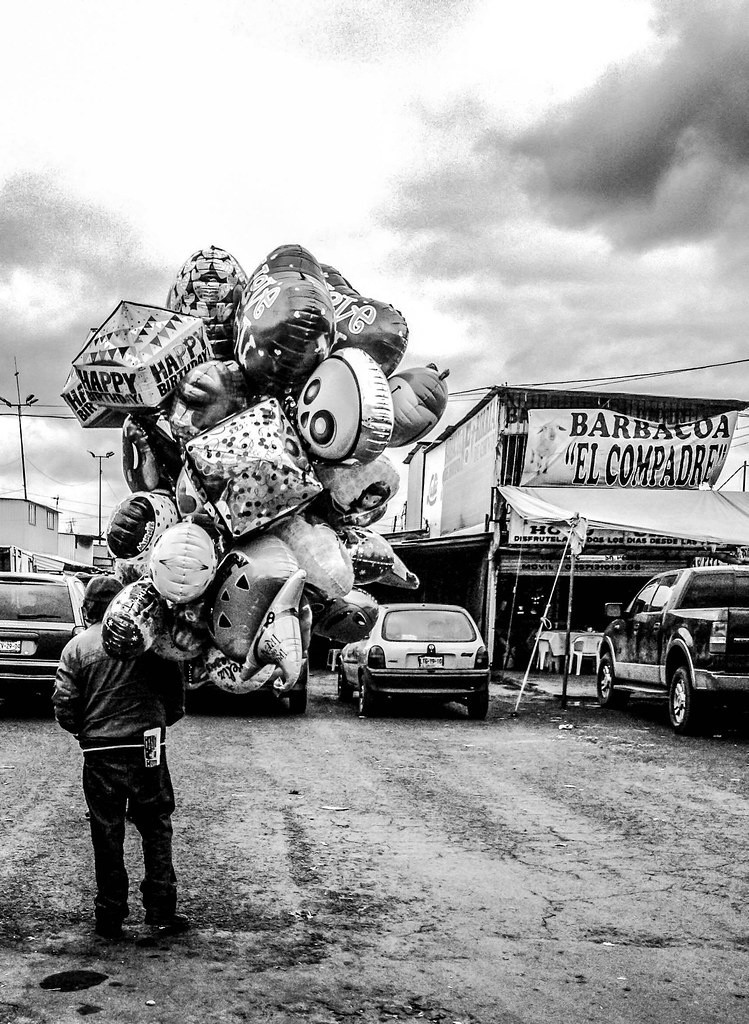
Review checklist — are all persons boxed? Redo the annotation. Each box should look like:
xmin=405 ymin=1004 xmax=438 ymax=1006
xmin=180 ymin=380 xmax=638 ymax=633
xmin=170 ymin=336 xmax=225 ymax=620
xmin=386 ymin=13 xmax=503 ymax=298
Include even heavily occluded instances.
xmin=53 ymin=575 xmax=188 ymax=939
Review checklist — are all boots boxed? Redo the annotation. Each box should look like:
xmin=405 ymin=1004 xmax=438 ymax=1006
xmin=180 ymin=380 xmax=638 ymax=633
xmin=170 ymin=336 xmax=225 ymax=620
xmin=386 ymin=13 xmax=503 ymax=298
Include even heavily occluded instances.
xmin=95 ymin=896 xmax=139 ymax=940
xmin=141 ymin=880 xmax=188 ymax=925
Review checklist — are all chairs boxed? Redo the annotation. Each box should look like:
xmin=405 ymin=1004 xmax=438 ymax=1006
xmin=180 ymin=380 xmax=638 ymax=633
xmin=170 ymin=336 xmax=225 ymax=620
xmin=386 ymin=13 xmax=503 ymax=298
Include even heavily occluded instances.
xmin=567 ymin=634 xmax=603 ymax=676
xmin=428 ymin=621 xmax=447 ymax=639
xmin=387 ymin=622 xmax=402 ymax=640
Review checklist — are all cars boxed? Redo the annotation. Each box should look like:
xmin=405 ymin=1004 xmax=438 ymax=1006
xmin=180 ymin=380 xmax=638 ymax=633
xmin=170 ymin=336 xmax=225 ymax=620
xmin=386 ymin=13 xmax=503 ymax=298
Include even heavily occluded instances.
xmin=338 ymin=601 xmax=491 ymax=719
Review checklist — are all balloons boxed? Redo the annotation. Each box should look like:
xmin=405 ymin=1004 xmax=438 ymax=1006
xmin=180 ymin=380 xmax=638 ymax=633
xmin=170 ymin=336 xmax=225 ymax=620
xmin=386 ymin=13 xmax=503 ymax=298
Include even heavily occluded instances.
xmin=60 ymin=244 xmax=450 ymax=695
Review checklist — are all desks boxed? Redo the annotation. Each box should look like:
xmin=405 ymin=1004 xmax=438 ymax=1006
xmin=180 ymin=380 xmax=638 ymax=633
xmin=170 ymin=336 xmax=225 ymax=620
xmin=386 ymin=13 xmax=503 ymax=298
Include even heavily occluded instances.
xmin=534 ymin=631 xmax=604 ymax=674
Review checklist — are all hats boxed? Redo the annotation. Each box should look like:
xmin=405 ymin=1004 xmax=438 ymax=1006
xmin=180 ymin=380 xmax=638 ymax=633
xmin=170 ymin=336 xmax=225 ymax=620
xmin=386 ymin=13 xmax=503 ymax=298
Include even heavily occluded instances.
xmin=83 ymin=578 xmax=124 ymax=621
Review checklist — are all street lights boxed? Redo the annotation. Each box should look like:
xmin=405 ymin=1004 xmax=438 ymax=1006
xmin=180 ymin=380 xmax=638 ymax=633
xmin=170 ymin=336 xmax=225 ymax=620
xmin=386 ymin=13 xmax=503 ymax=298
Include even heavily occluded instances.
xmin=87 ymin=450 xmax=115 ymax=546
xmin=0 ymin=394 xmax=38 ymax=500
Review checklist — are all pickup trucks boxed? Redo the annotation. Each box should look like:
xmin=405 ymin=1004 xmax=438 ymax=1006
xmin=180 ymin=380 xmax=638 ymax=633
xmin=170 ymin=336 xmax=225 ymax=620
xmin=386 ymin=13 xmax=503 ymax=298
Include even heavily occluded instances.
xmin=595 ymin=566 xmax=748 ymax=733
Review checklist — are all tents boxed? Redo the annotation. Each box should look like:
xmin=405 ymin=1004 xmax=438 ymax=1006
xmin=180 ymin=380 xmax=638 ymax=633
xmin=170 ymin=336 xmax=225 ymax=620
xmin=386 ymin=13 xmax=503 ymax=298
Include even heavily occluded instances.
xmin=497 ymin=485 xmax=749 ymax=710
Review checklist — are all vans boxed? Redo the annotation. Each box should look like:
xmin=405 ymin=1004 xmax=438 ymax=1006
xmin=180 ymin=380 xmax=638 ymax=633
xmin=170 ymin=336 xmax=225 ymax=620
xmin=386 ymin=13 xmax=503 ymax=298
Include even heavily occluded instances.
xmin=1 ymin=568 xmax=92 ymax=711
